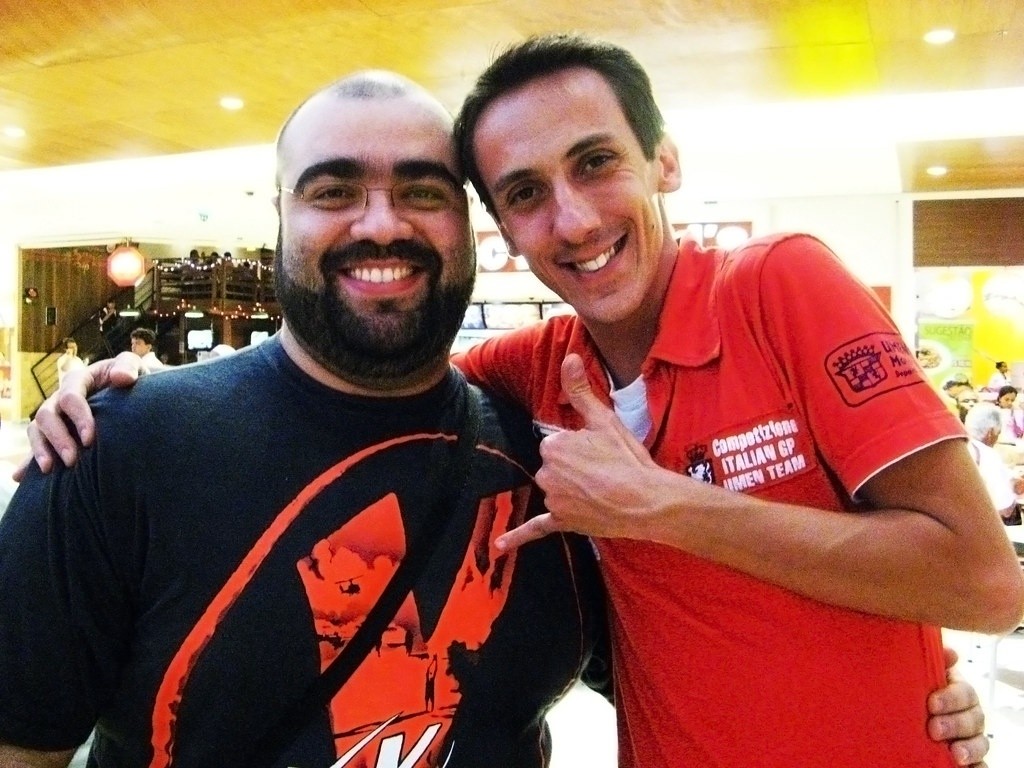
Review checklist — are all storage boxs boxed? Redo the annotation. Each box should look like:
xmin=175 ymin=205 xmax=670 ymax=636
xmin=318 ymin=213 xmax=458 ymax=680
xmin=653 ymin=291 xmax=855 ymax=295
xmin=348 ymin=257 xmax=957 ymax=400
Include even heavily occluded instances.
xmin=460 ymin=303 xmax=576 ymax=331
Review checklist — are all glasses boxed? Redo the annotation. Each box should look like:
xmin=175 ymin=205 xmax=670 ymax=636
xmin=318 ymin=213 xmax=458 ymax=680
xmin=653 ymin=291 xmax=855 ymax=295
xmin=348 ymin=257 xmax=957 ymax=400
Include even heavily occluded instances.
xmin=278 ymin=180 xmax=464 ymax=218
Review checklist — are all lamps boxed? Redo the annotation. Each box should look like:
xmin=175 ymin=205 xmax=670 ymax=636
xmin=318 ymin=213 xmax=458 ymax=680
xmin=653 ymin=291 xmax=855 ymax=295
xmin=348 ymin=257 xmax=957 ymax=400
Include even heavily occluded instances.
xmin=105 ymin=236 xmax=147 ymax=287
xmin=22 ymin=288 xmax=38 ymax=305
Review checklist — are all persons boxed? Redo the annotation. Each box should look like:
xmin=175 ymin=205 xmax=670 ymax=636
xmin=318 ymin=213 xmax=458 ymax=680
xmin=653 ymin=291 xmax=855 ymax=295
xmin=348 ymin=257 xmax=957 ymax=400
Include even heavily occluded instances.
xmin=98 ymin=299 xmax=116 ymax=334
xmin=57 ymin=338 xmax=83 ymax=388
xmin=942 ymin=361 xmax=1024 ymax=526
xmin=131 ymin=328 xmax=162 ymax=373
xmin=0 ymin=71 xmax=991 ymax=768
xmin=10 ymin=37 xmax=1024 ymax=768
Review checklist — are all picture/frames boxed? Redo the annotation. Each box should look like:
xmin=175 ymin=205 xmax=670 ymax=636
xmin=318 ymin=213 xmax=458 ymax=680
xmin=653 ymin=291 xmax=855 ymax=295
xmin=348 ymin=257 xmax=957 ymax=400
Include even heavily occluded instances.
xmin=46 ymin=306 xmax=57 ymax=326
